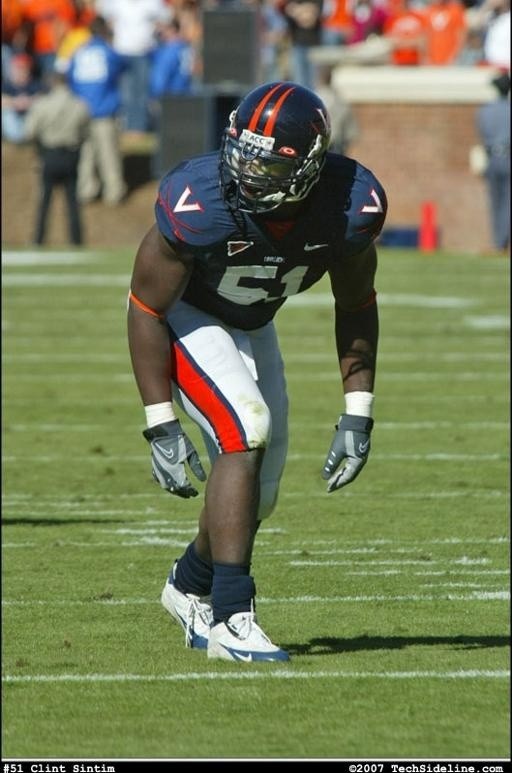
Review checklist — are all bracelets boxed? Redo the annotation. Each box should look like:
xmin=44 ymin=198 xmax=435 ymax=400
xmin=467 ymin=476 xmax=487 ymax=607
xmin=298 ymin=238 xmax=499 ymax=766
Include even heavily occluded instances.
xmin=143 ymin=400 xmax=178 ymax=427
xmin=342 ymin=389 xmax=377 ymax=420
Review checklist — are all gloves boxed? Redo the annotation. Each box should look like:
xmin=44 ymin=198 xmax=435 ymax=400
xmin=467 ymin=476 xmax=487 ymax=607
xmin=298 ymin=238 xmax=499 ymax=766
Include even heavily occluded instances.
xmin=142 ymin=417 xmax=206 ymax=499
xmin=323 ymin=414 xmax=374 ymax=492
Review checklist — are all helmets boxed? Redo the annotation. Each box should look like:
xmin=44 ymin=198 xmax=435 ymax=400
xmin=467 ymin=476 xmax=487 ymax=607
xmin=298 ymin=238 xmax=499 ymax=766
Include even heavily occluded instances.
xmin=217 ymin=83 xmax=331 ymax=214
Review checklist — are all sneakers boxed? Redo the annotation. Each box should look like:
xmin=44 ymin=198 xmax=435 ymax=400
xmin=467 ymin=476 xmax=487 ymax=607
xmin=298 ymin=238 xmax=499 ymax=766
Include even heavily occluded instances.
xmin=160 ymin=560 xmax=213 ymax=650
xmin=206 ymin=612 xmax=289 ymax=662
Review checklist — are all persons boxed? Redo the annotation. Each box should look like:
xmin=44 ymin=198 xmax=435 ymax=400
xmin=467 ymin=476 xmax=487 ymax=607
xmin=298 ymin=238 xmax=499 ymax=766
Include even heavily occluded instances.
xmin=21 ymin=69 xmax=91 ymax=246
xmin=125 ymin=79 xmax=390 ymax=662
xmin=474 ymin=71 xmax=511 ymax=249
xmin=72 ymin=17 xmax=131 ymax=206
xmin=0 ymin=0 xmax=512 ymax=136
xmin=310 ymin=67 xmax=360 ymax=157
